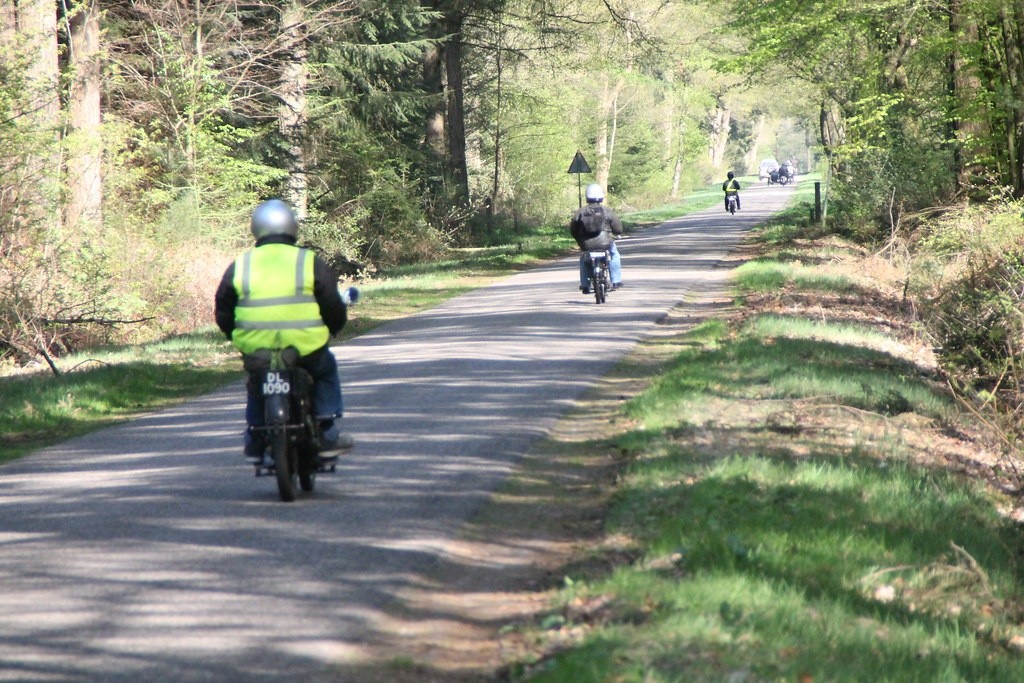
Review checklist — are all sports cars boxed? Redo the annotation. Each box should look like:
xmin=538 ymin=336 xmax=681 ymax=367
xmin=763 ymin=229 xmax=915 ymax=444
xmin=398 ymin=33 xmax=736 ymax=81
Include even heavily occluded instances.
xmin=767 ymin=175 xmax=787 ymax=186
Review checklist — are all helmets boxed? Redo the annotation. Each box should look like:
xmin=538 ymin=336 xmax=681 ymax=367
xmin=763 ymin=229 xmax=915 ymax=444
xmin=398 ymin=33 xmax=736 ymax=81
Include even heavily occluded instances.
xmin=586 ymin=183 xmax=603 ymax=203
xmin=250 ymin=199 xmax=298 ymax=240
xmin=727 ymin=172 xmax=734 ymax=178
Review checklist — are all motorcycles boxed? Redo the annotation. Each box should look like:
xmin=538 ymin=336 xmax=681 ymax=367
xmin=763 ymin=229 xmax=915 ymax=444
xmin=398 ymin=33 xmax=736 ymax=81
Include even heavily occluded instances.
xmin=582 ymin=248 xmax=617 ymax=303
xmin=727 ymin=195 xmax=736 ymax=214
xmin=247 ymin=366 xmax=338 ymax=501
xmin=788 ymin=175 xmax=794 ymax=184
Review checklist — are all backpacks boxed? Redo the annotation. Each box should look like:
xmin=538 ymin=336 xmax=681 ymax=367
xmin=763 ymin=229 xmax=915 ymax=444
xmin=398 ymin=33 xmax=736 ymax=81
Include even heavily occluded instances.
xmin=578 ymin=206 xmax=603 ymax=236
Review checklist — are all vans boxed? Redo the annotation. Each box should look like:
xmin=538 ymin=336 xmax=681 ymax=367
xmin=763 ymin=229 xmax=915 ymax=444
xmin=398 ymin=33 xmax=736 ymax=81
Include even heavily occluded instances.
xmin=760 ymin=159 xmax=778 ymax=179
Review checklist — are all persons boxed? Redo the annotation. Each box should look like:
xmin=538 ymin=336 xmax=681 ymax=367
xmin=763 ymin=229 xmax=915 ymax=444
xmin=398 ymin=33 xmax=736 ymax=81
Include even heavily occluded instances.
xmin=571 ymin=184 xmax=623 ymax=294
xmin=215 ymin=200 xmax=353 ymax=458
xmin=723 ymin=172 xmax=740 ymax=210
xmin=779 ymin=160 xmax=793 ymax=181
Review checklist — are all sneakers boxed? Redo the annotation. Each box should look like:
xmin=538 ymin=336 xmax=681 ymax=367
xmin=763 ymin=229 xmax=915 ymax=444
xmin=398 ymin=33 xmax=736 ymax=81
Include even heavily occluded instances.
xmin=579 ymin=285 xmax=590 ymax=295
xmin=317 ymin=434 xmax=355 ymax=458
xmin=244 ymin=446 xmax=265 ymax=463
xmin=612 ymin=282 xmax=624 ymax=289
